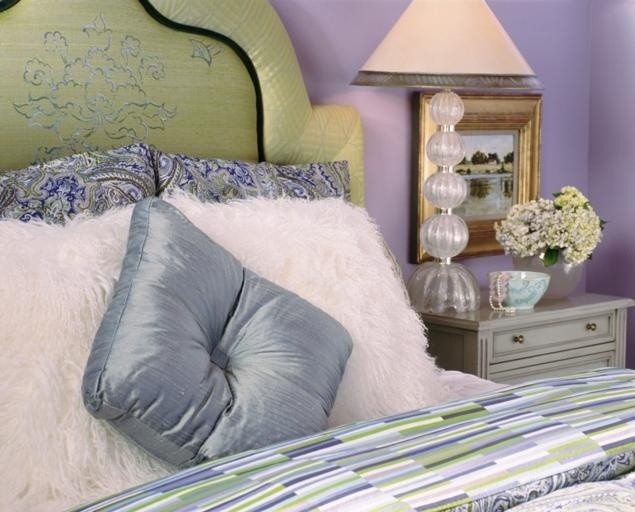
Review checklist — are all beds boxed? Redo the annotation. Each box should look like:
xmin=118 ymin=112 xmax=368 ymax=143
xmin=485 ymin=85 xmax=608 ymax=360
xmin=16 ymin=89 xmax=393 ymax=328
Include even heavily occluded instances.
xmin=0 ymin=2 xmax=635 ymax=512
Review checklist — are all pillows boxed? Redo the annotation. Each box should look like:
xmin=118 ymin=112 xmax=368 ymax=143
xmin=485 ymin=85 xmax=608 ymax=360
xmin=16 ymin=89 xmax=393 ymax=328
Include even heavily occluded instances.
xmin=150 ymin=144 xmax=350 ymax=211
xmin=80 ymin=194 xmax=356 ymax=471
xmin=1 ymin=202 xmax=186 ymax=509
xmin=1 ymin=146 xmax=160 ymax=232
xmin=160 ymin=184 xmax=463 ymax=430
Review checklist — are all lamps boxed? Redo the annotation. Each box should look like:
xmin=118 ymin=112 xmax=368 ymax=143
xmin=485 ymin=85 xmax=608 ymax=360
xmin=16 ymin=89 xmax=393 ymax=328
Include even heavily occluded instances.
xmin=349 ymin=0 xmax=544 ymax=314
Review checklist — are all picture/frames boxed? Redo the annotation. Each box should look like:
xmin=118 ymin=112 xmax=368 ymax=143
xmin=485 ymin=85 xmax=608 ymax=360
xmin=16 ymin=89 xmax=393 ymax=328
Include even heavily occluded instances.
xmin=413 ymin=91 xmax=543 ymax=266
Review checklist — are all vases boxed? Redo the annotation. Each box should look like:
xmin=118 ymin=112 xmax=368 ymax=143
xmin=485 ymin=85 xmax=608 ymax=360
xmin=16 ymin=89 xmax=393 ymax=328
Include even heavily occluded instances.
xmin=512 ymin=254 xmax=585 ymax=301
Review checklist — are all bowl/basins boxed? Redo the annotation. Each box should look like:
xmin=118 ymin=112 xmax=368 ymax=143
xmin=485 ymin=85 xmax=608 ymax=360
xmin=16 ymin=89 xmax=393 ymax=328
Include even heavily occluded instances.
xmin=488 ymin=270 xmax=551 ymax=310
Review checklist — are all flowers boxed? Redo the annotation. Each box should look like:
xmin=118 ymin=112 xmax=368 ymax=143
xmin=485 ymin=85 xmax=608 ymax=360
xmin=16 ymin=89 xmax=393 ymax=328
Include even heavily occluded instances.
xmin=492 ymin=183 xmax=607 ymax=266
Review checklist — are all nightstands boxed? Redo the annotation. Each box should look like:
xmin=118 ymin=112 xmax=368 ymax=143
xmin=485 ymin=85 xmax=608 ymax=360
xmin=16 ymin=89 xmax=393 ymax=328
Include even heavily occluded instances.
xmin=417 ymin=294 xmax=635 ymax=386
